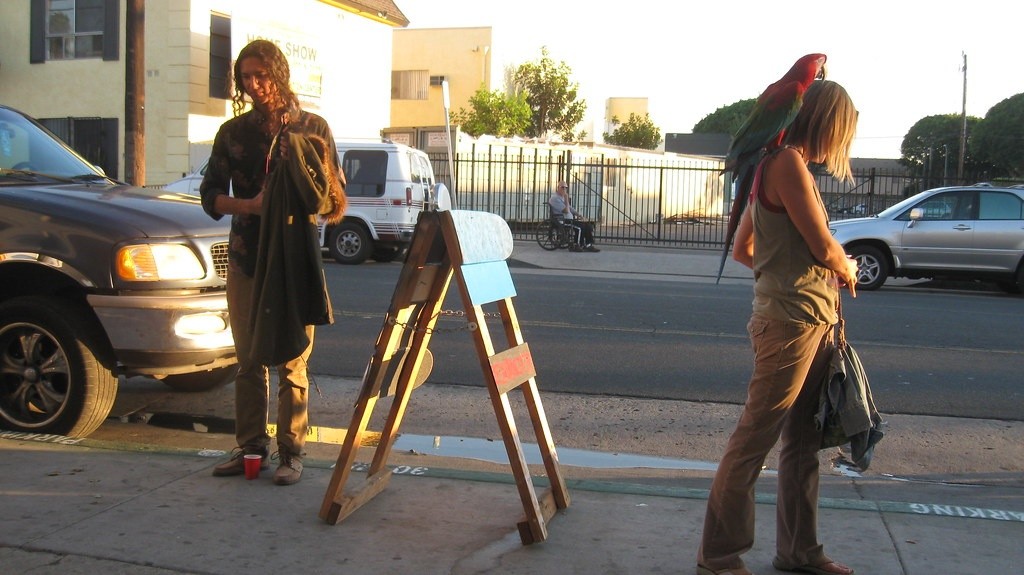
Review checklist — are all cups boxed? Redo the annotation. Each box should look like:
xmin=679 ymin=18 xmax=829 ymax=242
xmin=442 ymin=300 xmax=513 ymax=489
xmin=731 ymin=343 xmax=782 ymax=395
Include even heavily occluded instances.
xmin=837 ymin=255 xmax=852 ymax=288
xmin=244 ymin=454 xmax=262 ymax=479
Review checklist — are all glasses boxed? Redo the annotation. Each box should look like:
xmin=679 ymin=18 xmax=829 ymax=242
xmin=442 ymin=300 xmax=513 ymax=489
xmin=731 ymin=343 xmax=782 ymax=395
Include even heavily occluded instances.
xmin=559 ymin=185 xmax=568 ymax=189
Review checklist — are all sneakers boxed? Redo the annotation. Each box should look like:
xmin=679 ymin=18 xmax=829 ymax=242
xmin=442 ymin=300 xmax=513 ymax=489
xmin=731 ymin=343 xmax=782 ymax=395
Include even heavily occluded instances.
xmin=270 ymin=443 xmax=305 ymax=486
xmin=213 ymin=447 xmax=268 ymax=474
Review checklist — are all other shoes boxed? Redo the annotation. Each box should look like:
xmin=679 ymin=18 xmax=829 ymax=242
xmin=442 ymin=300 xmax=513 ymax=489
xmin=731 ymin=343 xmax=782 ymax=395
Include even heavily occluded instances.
xmin=583 ymin=245 xmax=600 ymax=252
xmin=568 ymin=244 xmax=587 ymax=252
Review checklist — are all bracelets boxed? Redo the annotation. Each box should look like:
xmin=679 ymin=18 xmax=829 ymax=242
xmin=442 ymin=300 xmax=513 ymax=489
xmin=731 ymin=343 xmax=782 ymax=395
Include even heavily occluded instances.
xmin=578 ymin=215 xmax=581 ymax=217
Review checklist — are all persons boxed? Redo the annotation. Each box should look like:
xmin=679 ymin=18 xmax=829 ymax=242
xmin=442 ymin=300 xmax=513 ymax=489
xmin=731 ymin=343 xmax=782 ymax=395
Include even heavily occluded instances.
xmin=200 ymin=39 xmax=349 ymax=486
xmin=695 ymin=79 xmax=860 ymax=575
xmin=549 ymin=180 xmax=601 ymax=252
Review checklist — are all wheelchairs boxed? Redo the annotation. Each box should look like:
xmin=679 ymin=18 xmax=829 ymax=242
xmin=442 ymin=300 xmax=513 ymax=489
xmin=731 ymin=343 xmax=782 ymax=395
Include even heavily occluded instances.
xmin=536 ymin=202 xmax=586 ymax=251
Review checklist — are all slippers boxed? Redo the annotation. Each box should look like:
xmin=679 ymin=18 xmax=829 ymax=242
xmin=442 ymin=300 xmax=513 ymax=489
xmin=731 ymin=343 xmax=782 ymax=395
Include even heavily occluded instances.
xmin=696 ymin=554 xmax=752 ymax=575
xmin=772 ymin=551 xmax=855 ymax=575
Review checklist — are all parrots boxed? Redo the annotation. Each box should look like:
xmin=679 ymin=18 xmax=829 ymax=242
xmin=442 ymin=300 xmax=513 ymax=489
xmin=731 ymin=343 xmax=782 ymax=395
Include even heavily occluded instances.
xmin=715 ymin=53 xmax=827 ymax=285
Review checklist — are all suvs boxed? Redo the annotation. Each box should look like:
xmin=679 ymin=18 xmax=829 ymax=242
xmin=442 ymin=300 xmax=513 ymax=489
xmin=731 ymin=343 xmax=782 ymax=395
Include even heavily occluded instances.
xmin=823 ymin=182 xmax=1024 ymax=291
xmin=160 ymin=137 xmax=452 ymax=264
xmin=0 ymin=104 xmax=240 ymax=441
